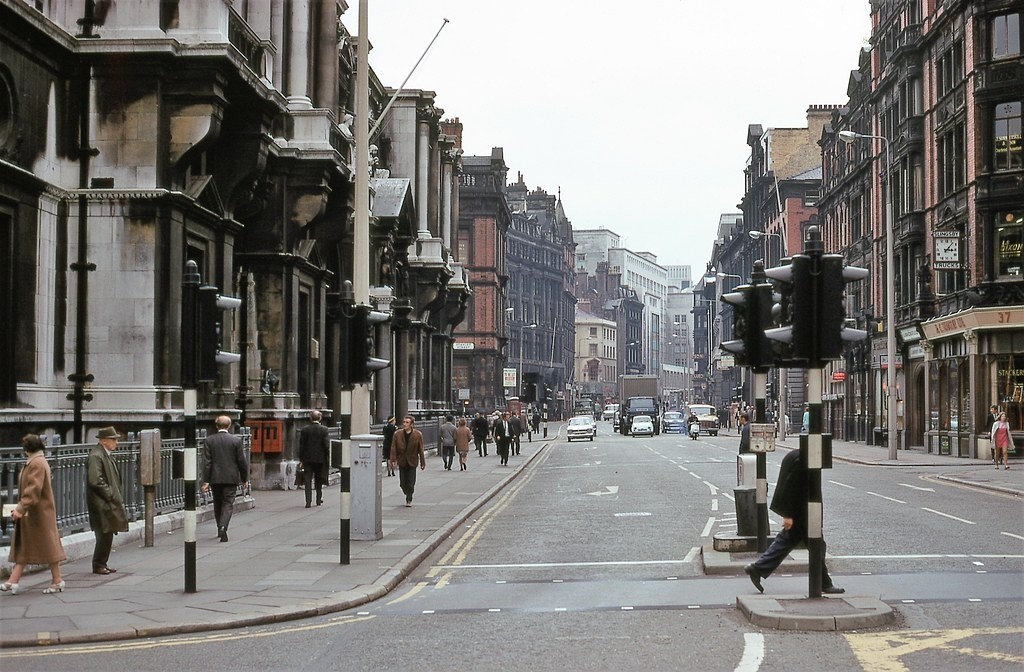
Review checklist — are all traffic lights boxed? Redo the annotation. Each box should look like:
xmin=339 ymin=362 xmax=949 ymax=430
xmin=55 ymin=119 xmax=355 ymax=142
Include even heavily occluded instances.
xmin=544 ymin=384 xmax=552 ymax=401
xmin=765 ymin=382 xmax=776 ymax=400
xmin=718 ymin=232 xmax=869 ymax=374
xmin=732 ymin=381 xmax=747 ymax=403
xmin=343 ymin=301 xmax=392 ymax=387
xmin=198 ymin=284 xmax=241 ymax=385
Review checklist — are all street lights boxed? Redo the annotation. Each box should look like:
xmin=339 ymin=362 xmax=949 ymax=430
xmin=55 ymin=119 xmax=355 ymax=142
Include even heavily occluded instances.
xmin=520 ymin=324 xmax=537 ymax=396
xmin=749 ymin=230 xmax=785 ymax=442
xmin=716 ymin=272 xmax=746 ymax=390
xmin=668 ymin=321 xmax=690 ymax=409
xmin=838 ymin=131 xmax=898 ymax=460
xmin=578 ymin=337 xmax=592 ymax=399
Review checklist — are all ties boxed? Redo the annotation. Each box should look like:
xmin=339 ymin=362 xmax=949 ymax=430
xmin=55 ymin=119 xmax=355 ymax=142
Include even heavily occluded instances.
xmin=995 ymin=414 xmax=996 ymax=419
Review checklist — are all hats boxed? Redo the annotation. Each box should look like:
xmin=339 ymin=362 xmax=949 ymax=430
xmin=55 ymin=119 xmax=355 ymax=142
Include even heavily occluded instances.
xmin=95 ymin=426 xmax=121 ymax=438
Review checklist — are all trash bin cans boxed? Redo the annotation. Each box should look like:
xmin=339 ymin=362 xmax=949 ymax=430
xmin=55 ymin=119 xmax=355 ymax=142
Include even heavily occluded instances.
xmin=978 ymin=433 xmax=993 ymax=459
xmin=733 ymin=456 xmax=771 ymax=535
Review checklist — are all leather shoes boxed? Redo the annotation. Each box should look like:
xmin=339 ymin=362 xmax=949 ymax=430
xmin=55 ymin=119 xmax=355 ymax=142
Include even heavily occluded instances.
xmin=743 ymin=565 xmax=763 ymax=593
xmin=822 ymin=585 xmax=845 ymax=593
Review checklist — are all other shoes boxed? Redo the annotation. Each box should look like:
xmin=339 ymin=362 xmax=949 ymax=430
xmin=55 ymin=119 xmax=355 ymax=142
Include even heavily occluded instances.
xmin=317 ymin=501 xmax=323 ymax=506
xmin=405 ymin=500 xmax=412 ymax=507
xmin=995 ymin=464 xmax=999 ymax=469
xmin=105 ymin=566 xmax=116 ymax=573
xmin=218 ymin=526 xmax=228 ymax=542
xmin=1005 ymin=466 xmax=1009 ymax=470
xmin=92 ymin=566 xmax=111 ymax=574
xmin=306 ymin=501 xmax=311 ymax=507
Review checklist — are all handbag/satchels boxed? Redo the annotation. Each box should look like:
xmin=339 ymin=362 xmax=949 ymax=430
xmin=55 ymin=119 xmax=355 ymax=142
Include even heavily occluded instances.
xmin=294 ymin=463 xmax=304 ymax=484
xmin=2 ymin=504 xmax=28 ymax=517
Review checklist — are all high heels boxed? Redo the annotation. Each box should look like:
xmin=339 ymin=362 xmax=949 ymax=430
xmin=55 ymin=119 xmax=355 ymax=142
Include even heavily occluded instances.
xmin=0 ymin=583 xmax=19 ymax=595
xmin=43 ymin=580 xmax=65 ymax=594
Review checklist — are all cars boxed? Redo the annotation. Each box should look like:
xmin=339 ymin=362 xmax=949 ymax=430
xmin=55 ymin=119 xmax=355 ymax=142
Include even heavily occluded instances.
xmin=603 ymin=374 xmax=662 ymax=438
xmin=567 ymin=398 xmax=603 ymax=442
xmin=662 ymin=404 xmax=720 ymax=437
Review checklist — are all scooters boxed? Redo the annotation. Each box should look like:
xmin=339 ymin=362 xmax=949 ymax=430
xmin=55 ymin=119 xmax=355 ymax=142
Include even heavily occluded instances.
xmin=689 ymin=421 xmax=702 ymax=439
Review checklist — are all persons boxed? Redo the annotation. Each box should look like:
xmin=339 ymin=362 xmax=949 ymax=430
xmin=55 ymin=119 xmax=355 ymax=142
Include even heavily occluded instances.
xmin=987 ymin=406 xmax=999 ymax=435
xmin=990 ymin=411 xmax=1016 ymax=470
xmin=454 ymin=417 xmax=472 ymax=471
xmin=687 ymin=414 xmax=700 ymax=437
xmin=744 ymin=448 xmax=845 ymax=593
xmin=0 ymin=434 xmax=66 ymax=595
xmin=472 ymin=412 xmax=490 ymax=457
xmin=201 ymin=416 xmax=248 ymax=542
xmin=803 ymin=407 xmax=809 ymax=430
xmin=495 ymin=410 xmax=515 ymax=466
xmin=298 ymin=411 xmax=331 ymax=508
xmin=383 ymin=415 xmax=398 ymax=476
xmin=716 ymin=402 xmax=771 ymax=428
xmin=490 ymin=409 xmax=527 ymax=456
xmin=778 ymin=413 xmax=789 ymax=438
xmin=390 ymin=415 xmax=426 ymax=507
xmin=532 ymin=412 xmax=541 ymax=434
xmin=738 ymin=413 xmax=755 ymax=454
xmin=87 ymin=426 xmax=129 ymax=574
xmin=439 ymin=416 xmax=457 ymax=470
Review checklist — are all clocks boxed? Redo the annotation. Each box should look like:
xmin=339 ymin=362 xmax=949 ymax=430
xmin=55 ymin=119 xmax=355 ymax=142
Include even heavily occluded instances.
xmin=933 ymin=236 xmax=962 ymax=263
